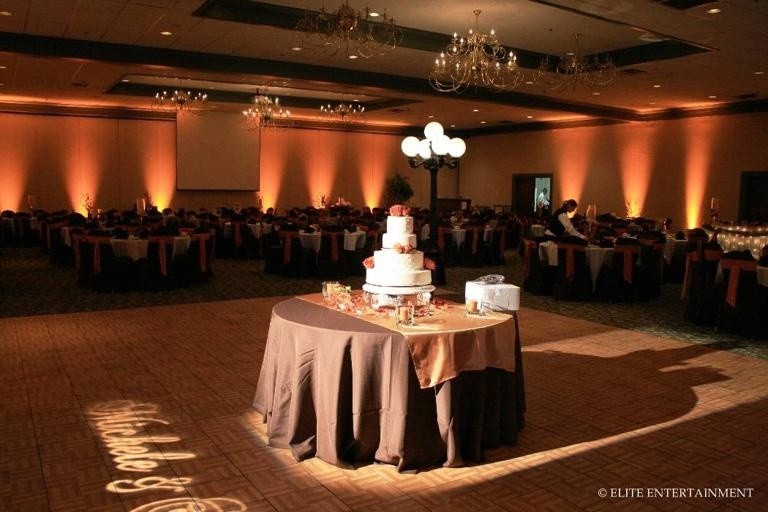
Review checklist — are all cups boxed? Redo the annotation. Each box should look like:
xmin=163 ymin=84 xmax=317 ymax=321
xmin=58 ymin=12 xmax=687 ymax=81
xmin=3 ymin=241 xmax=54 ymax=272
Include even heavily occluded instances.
xmin=395 ymin=305 xmax=414 ymax=328
xmin=465 ymin=296 xmax=483 ymax=315
xmin=321 ymin=281 xmax=339 ymax=304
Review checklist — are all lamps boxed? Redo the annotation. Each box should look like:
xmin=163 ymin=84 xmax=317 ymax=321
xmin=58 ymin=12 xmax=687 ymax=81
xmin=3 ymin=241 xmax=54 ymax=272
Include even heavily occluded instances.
xmin=242 ymin=95 xmax=294 ymax=132
xmin=150 ymin=87 xmax=211 ymax=117
xmin=429 ymin=9 xmax=525 ymax=92
xmin=531 ymin=31 xmax=619 ymax=92
xmin=290 ymin=1 xmax=403 ymax=60
xmin=320 ymin=94 xmax=367 ymax=129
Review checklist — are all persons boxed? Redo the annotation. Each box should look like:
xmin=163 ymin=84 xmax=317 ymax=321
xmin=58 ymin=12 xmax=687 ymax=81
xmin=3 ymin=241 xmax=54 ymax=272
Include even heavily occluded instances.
xmin=537 ymin=188 xmax=550 ymax=217
xmin=542 ymin=198 xmax=592 ymax=245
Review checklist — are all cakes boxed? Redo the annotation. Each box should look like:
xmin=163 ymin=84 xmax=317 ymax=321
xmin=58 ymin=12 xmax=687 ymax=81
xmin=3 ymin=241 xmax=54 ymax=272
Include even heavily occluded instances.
xmin=362 ymin=204 xmax=436 ymax=287
